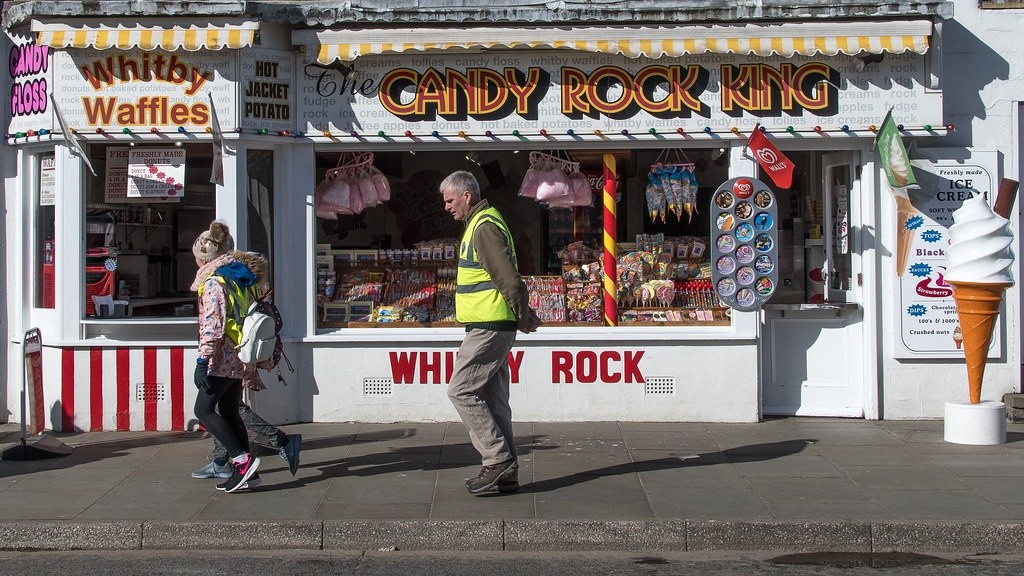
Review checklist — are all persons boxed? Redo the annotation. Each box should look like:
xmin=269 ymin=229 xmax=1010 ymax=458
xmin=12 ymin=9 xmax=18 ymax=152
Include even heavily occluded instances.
xmin=439 ymin=170 xmax=542 ymax=496
xmin=191 ymin=221 xmax=302 ymax=492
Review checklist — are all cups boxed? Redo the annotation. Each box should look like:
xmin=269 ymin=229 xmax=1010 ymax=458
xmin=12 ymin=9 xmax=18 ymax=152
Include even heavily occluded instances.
xmin=318 ymin=272 xmax=336 ymax=297
xmin=810 ymin=225 xmax=816 ymax=239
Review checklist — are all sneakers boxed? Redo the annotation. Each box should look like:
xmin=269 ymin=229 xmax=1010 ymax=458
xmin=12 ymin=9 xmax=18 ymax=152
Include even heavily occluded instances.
xmin=215 ymin=472 xmax=262 ymax=490
xmin=280 ymin=433 xmax=302 ymax=477
xmin=191 ymin=459 xmax=233 ymax=479
xmin=465 ymin=478 xmax=519 ymax=491
xmin=227 ymin=453 xmax=260 ymax=492
xmin=466 ymin=459 xmax=516 ymax=493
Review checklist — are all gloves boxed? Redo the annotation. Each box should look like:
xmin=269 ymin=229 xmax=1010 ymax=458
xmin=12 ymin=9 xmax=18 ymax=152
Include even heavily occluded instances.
xmin=194 ymin=360 xmax=210 ymax=388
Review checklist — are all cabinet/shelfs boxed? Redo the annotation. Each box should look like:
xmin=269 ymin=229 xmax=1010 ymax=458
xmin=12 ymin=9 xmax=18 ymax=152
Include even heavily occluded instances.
xmin=87 ymin=204 xmax=214 ymax=314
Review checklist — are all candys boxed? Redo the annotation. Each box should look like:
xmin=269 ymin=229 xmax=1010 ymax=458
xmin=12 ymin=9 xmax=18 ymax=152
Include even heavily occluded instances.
xmin=616 ymin=250 xmax=675 ymax=308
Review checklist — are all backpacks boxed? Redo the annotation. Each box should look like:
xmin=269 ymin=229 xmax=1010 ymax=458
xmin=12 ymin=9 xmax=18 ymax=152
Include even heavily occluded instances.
xmin=206 ymin=276 xmax=282 ymax=370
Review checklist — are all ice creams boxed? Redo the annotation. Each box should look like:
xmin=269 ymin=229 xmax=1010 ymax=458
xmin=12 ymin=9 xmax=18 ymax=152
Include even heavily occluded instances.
xmin=886 ymin=133 xmax=940 ymax=277
xmin=942 ymin=179 xmax=1020 ymax=405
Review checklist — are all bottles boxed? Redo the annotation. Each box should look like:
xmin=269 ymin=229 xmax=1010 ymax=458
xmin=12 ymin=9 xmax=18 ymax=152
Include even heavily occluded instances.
xmin=550 ymin=209 xmax=558 ymax=228
xmin=547 ymin=256 xmax=562 ymax=275
xmin=130 ymin=206 xmax=144 ymax=222
xmin=151 ymin=207 xmax=166 ymax=224
xmin=831 ymin=272 xmax=839 ymax=290
xmin=119 ymin=278 xmax=130 ymax=299
xmin=551 ymin=238 xmax=573 ymax=261
xmin=558 ymin=209 xmax=566 ymax=228
xmin=848 ymin=276 xmax=852 ymax=290
xmin=567 ymin=208 xmax=574 ymax=228
xmin=46 ymin=236 xmax=53 ymax=263
xmin=586 ymin=235 xmax=603 ymax=259
xmin=583 ymin=209 xmax=590 ymax=230
xmin=598 ymin=211 xmax=604 ymax=231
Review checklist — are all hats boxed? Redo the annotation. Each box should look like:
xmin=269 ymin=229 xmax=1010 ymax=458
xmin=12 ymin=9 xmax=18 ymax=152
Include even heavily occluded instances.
xmin=234 ymin=249 xmax=272 ymax=301
xmin=191 ymin=223 xmax=235 ymax=264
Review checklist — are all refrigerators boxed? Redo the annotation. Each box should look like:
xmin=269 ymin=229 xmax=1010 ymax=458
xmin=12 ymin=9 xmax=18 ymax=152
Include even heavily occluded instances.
xmin=540 ymin=161 xmax=627 ymax=277
